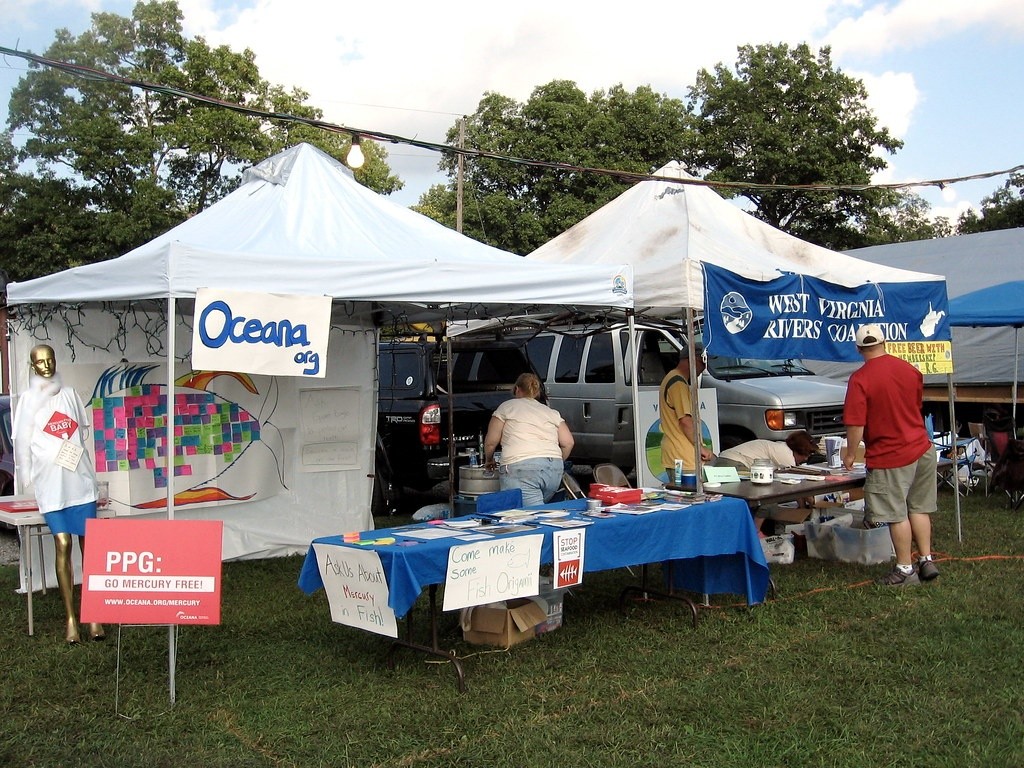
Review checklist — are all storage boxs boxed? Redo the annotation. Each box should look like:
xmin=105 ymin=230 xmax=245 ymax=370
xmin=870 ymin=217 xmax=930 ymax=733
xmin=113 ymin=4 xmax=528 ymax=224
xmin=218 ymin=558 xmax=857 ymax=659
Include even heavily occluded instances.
xmin=768 ymin=499 xmax=845 ymax=549
xmin=461 ymin=598 xmax=547 ymax=647
xmin=805 ymin=508 xmax=895 ymax=564
xmin=520 ymin=577 xmax=567 ymax=635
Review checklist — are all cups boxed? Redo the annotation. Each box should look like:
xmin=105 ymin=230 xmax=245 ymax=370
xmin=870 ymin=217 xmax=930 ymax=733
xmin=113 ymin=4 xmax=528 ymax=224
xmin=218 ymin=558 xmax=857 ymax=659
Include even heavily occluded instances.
xmin=586 ymin=500 xmax=602 ymax=510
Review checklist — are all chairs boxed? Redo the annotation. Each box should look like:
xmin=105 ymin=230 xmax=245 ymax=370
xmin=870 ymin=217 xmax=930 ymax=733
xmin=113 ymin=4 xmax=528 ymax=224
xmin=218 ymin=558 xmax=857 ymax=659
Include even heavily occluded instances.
xmin=594 ymin=463 xmax=633 ymax=489
xmin=924 ymin=414 xmax=978 ymax=497
xmin=640 ymin=348 xmax=667 ymax=384
xmin=988 ymin=438 xmax=1024 ymax=511
xmin=562 ymin=472 xmax=636 ymax=574
xmin=967 ymin=422 xmax=993 ymax=457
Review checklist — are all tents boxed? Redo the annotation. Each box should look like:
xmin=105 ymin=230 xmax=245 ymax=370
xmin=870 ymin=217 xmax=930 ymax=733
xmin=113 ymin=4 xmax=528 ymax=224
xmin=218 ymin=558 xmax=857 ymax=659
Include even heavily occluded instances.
xmin=447 ymin=158 xmax=963 ymax=609
xmin=6 ymin=143 xmax=650 ymax=709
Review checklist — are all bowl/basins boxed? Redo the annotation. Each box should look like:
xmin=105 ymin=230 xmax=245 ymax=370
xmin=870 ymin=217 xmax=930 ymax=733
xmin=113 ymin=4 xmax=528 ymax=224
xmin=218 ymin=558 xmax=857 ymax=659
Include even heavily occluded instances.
xmin=682 ymin=473 xmax=697 ymax=485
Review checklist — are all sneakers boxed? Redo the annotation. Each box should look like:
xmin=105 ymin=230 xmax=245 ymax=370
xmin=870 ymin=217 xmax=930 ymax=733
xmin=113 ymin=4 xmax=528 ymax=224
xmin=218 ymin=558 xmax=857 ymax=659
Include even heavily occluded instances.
xmin=914 ymin=560 xmax=940 ymax=580
xmin=873 ymin=563 xmax=921 ymax=586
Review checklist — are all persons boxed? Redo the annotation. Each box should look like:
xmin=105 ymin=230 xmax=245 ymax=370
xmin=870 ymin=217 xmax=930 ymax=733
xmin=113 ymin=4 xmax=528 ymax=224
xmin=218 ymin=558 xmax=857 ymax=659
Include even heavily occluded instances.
xmin=484 ymin=372 xmax=574 ymax=510
xmin=13 ymin=345 xmax=105 ymax=644
xmin=715 ymin=431 xmax=820 ymax=540
xmin=659 ymin=343 xmax=712 ymax=489
xmin=843 ymin=323 xmax=939 ymax=589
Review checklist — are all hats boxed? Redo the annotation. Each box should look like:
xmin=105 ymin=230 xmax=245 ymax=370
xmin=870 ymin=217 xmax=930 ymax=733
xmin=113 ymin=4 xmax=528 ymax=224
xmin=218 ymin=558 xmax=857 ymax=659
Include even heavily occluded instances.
xmin=856 ymin=324 xmax=884 ymax=347
xmin=679 ymin=342 xmax=704 ymax=358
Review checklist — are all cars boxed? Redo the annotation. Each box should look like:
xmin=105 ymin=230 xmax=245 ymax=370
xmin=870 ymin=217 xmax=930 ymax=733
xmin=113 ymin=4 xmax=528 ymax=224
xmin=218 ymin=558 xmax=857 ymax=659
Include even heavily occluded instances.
xmin=0 ymin=393 xmax=13 ymax=535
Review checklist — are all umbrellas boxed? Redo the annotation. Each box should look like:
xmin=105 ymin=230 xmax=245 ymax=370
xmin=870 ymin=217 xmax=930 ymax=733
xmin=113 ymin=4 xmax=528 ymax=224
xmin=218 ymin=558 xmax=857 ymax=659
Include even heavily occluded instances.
xmin=947 ymin=280 xmax=1024 ymax=445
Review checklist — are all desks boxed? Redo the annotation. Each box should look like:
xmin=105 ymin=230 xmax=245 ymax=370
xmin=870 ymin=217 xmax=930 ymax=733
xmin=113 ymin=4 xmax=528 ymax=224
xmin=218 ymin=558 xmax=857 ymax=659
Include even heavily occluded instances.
xmin=298 ymin=487 xmax=769 ymax=694
xmin=0 ymin=496 xmax=117 ymax=635
xmin=662 ymin=457 xmax=953 ymax=508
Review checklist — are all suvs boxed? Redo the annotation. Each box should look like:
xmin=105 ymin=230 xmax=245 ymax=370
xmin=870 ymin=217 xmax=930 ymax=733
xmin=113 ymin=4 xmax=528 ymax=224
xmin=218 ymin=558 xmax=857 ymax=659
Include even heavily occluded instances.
xmin=368 ymin=332 xmax=550 ymax=520
xmin=454 ymin=327 xmax=851 ymax=478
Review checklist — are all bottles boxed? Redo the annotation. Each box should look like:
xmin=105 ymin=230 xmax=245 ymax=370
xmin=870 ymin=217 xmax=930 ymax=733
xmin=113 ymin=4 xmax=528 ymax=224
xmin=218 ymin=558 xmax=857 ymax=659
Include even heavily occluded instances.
xmin=750 ymin=458 xmax=775 ymax=486
xmin=468 ymin=448 xmax=478 ymax=468
